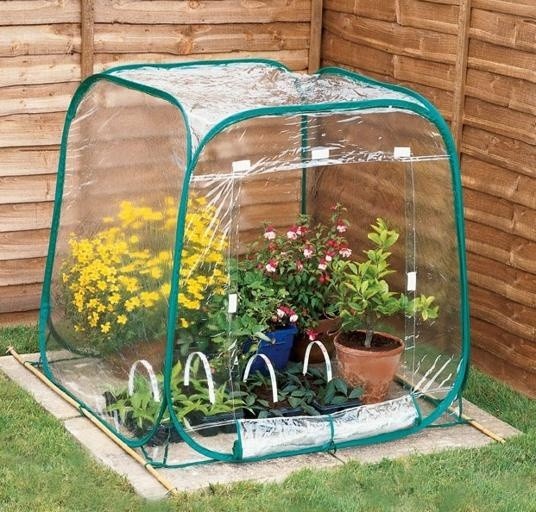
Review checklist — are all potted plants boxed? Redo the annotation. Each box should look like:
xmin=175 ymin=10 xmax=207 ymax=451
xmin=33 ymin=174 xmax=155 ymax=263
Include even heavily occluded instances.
xmin=326 ymin=217 xmax=440 ymax=404
xmin=196 ymin=258 xmax=298 ymax=377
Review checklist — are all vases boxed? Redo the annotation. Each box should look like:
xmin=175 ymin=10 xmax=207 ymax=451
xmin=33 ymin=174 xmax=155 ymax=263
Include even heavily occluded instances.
xmin=292 ymin=315 xmax=342 ymax=362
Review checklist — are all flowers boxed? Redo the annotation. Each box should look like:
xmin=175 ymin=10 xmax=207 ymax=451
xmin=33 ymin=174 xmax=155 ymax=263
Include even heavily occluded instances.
xmin=57 ymin=192 xmax=230 ymax=349
xmin=246 ymin=203 xmax=353 ymax=327
